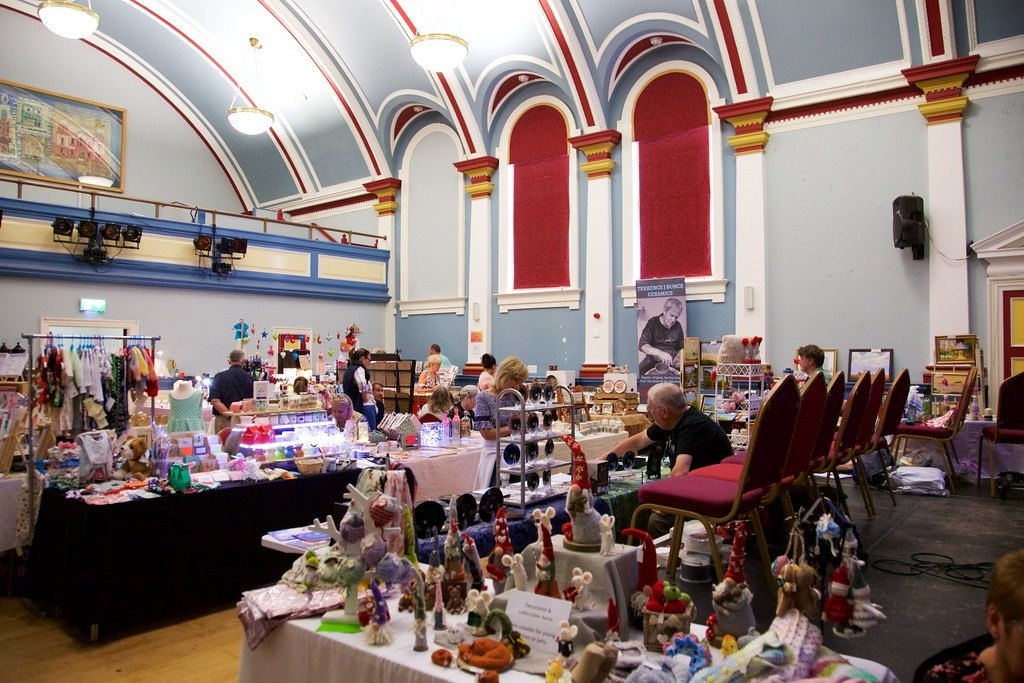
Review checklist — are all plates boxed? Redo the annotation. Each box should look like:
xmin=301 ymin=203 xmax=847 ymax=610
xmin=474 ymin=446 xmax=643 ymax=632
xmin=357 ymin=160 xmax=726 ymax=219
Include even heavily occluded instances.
xmin=412 ymin=382 xmax=636 ymax=538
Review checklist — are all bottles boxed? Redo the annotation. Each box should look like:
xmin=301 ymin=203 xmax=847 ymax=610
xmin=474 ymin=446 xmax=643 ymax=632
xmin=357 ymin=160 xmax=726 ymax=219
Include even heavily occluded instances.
xmin=461 ymin=411 xmax=470 ymax=438
xmin=448 ymin=417 xmax=452 ymax=436
xmin=452 ymin=408 xmax=461 ymax=441
xmin=942 ymin=394 xmax=979 ymax=421
xmin=317 ymin=455 xmax=357 ymax=473
xmin=442 ymin=412 xmax=449 ymax=443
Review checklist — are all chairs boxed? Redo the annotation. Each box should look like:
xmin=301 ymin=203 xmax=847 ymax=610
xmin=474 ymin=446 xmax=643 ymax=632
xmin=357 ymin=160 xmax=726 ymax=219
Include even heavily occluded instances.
xmin=669 ymin=370 xmax=828 ymax=578
xmin=888 ymin=365 xmax=980 ymax=494
xmin=816 ymin=369 xmax=875 ymax=522
xmin=832 ymin=367 xmax=912 ymax=508
xmin=625 ymin=371 xmax=804 ymax=591
xmin=976 ymin=371 xmax=1024 ymax=500
xmin=417 ymin=412 xmax=443 ymax=426
xmin=830 ymin=367 xmax=898 ymax=516
xmin=720 ymin=368 xmax=850 ymax=533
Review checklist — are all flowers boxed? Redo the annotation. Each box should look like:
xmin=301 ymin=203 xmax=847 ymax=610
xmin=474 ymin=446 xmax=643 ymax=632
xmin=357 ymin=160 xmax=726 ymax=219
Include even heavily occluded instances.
xmin=741 ymin=336 xmax=763 ymax=360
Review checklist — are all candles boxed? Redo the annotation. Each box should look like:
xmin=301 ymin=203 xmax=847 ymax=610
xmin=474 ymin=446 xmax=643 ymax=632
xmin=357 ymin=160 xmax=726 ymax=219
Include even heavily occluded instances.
xmin=985 ymin=408 xmax=993 ymax=417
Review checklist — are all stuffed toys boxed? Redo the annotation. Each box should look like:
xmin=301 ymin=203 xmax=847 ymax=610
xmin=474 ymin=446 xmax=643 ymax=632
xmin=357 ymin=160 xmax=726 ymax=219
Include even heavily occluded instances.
xmin=277 ymin=431 xmax=887 ymax=682
xmin=112 ymin=435 xmax=156 ymax=481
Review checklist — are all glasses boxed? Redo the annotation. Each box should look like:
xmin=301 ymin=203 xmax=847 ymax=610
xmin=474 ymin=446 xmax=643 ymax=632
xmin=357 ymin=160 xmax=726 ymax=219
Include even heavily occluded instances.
xmin=512 ymin=376 xmax=522 ymax=385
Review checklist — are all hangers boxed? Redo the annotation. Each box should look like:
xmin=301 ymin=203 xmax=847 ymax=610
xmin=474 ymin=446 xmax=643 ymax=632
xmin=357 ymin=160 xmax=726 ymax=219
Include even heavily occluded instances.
xmin=231 ymin=318 xmax=246 ymax=331
xmin=36 ymin=330 xmax=148 ymax=357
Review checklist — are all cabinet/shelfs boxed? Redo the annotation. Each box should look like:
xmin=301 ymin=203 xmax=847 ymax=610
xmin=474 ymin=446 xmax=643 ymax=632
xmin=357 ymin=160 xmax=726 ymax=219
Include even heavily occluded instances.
xmin=711 ymin=363 xmax=767 ymax=457
xmin=495 ymin=384 xmax=578 ymax=511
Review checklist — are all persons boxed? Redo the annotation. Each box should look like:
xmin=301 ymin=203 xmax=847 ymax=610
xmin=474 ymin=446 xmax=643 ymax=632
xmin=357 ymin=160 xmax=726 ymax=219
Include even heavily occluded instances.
xmin=470 ymin=356 xmax=530 ymax=490
xmin=207 ymin=350 xmax=259 ymax=435
xmin=327 ymin=393 xmax=371 ymax=433
xmin=414 ymin=344 xmax=557 ymax=430
xmin=638 ymin=298 xmax=684 ymax=375
xmin=371 ymin=383 xmax=385 ymax=431
xmin=595 ymin=383 xmax=730 ymax=539
xmin=799 ymin=344 xmax=834 ymax=389
xmin=293 ymin=376 xmax=308 ymax=394
xmin=912 ymin=549 xmax=1024 ymax=682
xmin=342 ymin=348 xmax=379 ymax=431
xmin=167 ymin=381 xmax=204 ymax=433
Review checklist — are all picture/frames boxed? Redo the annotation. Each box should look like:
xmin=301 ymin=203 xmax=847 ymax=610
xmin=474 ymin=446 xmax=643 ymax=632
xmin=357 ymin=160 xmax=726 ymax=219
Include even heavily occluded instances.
xmin=702 ymin=366 xmax=728 ymax=391
xmin=698 ymin=394 xmax=715 ymax=413
xmin=683 ymin=336 xmax=700 ymax=362
xmin=931 ymin=371 xmax=970 ymax=396
xmin=699 ymin=340 xmax=722 ymax=366
xmin=683 ymin=388 xmax=699 ymax=409
xmin=794 ymin=348 xmax=839 ymax=380
xmin=935 ymin=334 xmax=976 ymax=365
xmin=683 ymin=362 xmax=699 ymax=389
xmin=0 ymin=78 xmax=127 ymax=194
xmin=846 ymin=347 xmax=894 ymax=383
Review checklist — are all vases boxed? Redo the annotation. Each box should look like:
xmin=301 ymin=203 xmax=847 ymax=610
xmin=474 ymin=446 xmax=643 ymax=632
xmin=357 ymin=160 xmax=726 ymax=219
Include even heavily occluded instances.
xmin=742 ymin=348 xmax=762 ymax=364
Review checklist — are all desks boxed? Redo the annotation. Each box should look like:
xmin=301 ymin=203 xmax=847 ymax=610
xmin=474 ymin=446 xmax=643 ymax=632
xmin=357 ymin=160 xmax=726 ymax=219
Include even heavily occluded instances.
xmin=360 ymin=425 xmax=630 ymax=505
xmin=0 ymin=381 xmax=29 ymax=411
xmin=384 ymin=386 xmax=463 ymax=420
xmin=23 ymin=451 xmax=359 ymax=654
xmin=590 ymin=413 xmax=652 ymax=438
xmin=260 ymin=453 xmax=673 ymax=568
xmin=234 ymin=579 xmax=901 ymax=683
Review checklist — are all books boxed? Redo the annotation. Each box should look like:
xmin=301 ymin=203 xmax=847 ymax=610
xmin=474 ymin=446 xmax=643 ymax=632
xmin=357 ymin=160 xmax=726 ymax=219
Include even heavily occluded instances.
xmin=375 ymin=405 xmax=422 ymax=449
xmin=267 ymin=527 xmax=332 ymax=551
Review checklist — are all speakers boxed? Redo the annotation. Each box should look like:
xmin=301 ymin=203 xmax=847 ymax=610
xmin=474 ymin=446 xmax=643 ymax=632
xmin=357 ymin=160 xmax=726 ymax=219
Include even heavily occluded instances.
xmin=892 ymin=195 xmax=925 ymax=247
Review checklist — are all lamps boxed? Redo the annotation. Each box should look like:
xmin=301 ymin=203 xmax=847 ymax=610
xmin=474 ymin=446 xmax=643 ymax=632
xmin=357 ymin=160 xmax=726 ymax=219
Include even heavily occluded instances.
xmin=100 ymin=222 xmax=122 ymax=242
xmin=75 ymin=219 xmax=99 ymax=240
xmin=79 ymin=298 xmax=107 ymax=313
xmin=744 ymin=285 xmax=754 ymax=310
xmin=225 ymin=37 xmax=275 ymax=135
xmin=472 ymin=302 xmax=481 ymax=322
xmin=193 ymin=234 xmax=212 ymax=252
xmin=215 ymin=236 xmax=235 ymax=256
xmin=51 ymin=217 xmax=75 ymax=237
xmin=408 ymin=21 xmax=471 ymax=73
xmin=77 ymin=161 xmax=113 ymax=187
xmin=36 ymin=0 xmax=102 ymax=40
xmin=232 ymin=237 xmax=250 ymax=254
xmin=121 ymin=224 xmax=144 ymax=244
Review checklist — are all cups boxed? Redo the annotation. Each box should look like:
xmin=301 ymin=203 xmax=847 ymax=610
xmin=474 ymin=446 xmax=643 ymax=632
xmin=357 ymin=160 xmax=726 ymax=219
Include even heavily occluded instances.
xmin=376 ymin=440 xmax=400 ymax=454
